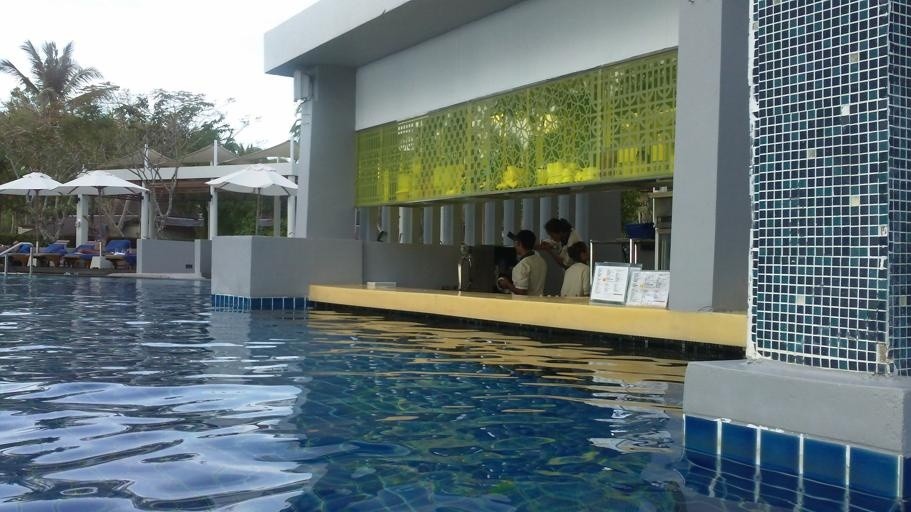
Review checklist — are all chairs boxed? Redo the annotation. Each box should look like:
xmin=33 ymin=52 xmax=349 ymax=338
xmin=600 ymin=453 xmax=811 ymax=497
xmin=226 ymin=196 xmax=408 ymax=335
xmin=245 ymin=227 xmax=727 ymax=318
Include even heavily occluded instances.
xmin=1 ymin=239 xmax=137 ymax=272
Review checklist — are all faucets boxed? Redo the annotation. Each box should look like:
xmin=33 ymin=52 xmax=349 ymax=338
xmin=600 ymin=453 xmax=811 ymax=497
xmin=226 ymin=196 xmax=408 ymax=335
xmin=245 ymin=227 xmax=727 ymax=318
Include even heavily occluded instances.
xmin=455 ymin=256 xmax=473 ymax=290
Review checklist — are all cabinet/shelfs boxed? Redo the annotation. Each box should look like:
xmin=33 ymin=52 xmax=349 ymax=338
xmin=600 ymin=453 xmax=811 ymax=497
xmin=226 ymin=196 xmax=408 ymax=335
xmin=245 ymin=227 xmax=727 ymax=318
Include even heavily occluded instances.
xmin=589 ymin=180 xmax=671 ymax=289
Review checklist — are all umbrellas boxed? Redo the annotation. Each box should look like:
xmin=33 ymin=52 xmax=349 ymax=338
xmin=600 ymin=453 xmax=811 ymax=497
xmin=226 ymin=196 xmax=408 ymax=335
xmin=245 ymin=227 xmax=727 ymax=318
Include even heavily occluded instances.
xmin=204 ymin=163 xmax=300 ymax=236
xmin=50 ymin=168 xmax=152 ymax=256
xmin=1 ymin=170 xmax=67 ymax=253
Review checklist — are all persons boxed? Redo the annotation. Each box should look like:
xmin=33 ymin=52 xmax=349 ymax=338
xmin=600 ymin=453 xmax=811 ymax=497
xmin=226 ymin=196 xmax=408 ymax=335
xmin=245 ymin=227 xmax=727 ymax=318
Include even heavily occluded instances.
xmin=558 ymin=239 xmax=591 ymax=298
xmin=540 ymin=218 xmax=587 ymax=267
xmin=493 ymin=228 xmax=548 ymax=296
